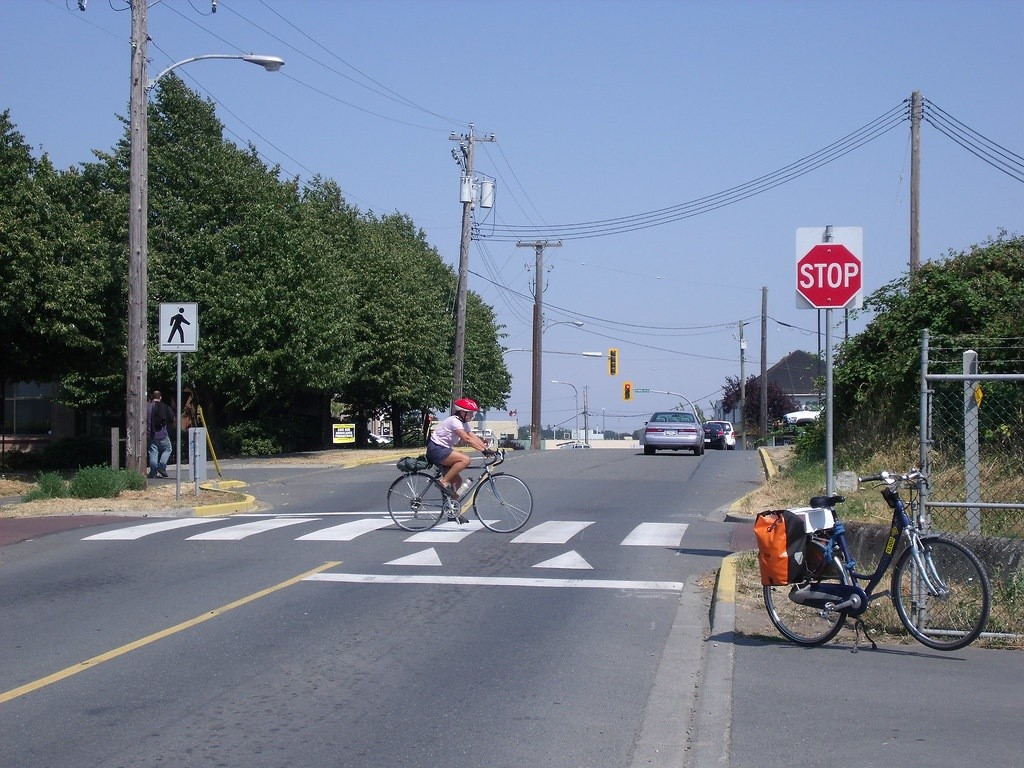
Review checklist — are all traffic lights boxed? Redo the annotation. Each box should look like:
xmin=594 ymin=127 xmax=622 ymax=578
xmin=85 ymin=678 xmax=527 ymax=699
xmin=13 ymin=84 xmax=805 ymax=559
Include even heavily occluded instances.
xmin=623 ymin=382 xmax=631 ymax=402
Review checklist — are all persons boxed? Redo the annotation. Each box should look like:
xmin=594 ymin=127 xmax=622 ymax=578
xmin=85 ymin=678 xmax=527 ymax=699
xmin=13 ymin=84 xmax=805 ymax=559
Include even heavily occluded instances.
xmin=147 ymin=390 xmax=177 ymax=478
xmin=426 ymin=397 xmax=498 ymax=524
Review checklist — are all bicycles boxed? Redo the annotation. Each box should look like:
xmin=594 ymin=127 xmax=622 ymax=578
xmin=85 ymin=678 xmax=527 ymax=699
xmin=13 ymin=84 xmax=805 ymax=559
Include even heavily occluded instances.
xmin=387 ymin=448 xmax=533 ymax=535
xmin=763 ymin=464 xmax=990 ymax=654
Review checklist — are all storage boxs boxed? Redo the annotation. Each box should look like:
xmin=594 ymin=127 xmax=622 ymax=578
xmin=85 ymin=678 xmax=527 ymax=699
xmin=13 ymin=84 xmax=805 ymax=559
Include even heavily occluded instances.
xmin=771 ymin=506 xmax=834 ymax=533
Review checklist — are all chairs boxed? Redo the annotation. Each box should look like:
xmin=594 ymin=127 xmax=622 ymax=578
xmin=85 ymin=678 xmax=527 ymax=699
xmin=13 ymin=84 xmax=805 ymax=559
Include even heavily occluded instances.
xmin=680 ymin=417 xmax=691 ymax=422
xmin=656 ymin=418 xmax=665 ymax=422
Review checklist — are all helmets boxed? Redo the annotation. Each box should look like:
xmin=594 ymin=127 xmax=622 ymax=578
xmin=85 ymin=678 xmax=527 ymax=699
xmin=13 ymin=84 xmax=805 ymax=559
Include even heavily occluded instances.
xmin=455 ymin=398 xmax=478 ymax=411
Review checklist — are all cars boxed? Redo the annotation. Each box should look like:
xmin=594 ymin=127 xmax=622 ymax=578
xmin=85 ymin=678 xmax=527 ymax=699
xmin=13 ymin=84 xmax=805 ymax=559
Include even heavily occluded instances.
xmin=369 ymin=433 xmax=393 ymax=443
xmin=643 ymin=411 xmax=704 ymax=456
xmin=783 ymin=406 xmax=823 ymax=432
xmin=703 ymin=421 xmax=736 ymax=450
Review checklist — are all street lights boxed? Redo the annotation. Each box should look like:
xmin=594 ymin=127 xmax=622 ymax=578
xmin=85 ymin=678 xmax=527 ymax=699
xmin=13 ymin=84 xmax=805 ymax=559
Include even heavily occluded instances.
xmin=531 ymin=321 xmax=584 ymax=450
xmin=551 ymin=380 xmax=579 ymax=443
xmin=125 ymin=54 xmax=287 ymax=470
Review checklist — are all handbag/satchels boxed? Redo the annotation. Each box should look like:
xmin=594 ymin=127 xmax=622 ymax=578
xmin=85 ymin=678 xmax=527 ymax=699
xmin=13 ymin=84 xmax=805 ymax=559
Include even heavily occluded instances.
xmin=397 ymin=454 xmax=430 ymax=473
xmin=755 ymin=508 xmax=832 ymax=587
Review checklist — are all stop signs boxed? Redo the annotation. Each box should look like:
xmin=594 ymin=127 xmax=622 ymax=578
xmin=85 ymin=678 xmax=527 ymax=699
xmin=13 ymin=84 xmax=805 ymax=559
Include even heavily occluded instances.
xmin=796 ymin=244 xmax=863 ymax=310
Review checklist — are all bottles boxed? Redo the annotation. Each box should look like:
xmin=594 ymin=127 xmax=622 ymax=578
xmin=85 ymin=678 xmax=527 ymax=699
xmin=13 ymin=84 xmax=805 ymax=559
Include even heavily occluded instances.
xmin=456 ymin=477 xmax=474 ymax=496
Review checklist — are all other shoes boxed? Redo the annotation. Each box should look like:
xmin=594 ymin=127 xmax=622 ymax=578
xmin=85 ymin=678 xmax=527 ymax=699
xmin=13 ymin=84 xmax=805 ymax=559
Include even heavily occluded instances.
xmin=147 ymin=472 xmax=157 ymax=478
xmin=438 ymin=480 xmax=456 ymax=496
xmin=158 ymin=469 xmax=168 ymax=477
xmin=448 ymin=515 xmax=469 ymax=523
